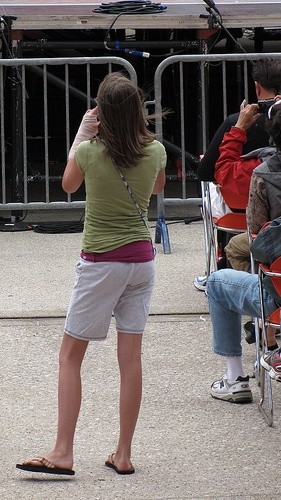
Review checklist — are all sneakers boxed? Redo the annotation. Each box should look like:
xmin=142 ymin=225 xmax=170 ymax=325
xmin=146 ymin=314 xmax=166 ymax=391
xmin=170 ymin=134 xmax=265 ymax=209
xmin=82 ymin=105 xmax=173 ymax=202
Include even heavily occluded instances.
xmin=260 ymin=351 xmax=281 ymax=382
xmin=194 ymin=276 xmax=207 ymax=291
xmin=210 ymin=375 xmax=253 ymax=403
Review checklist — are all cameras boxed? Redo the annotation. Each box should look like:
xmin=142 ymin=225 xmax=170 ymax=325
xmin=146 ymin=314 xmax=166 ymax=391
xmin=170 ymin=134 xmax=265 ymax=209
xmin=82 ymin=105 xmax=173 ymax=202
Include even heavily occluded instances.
xmin=258 ymin=99 xmax=275 ymax=114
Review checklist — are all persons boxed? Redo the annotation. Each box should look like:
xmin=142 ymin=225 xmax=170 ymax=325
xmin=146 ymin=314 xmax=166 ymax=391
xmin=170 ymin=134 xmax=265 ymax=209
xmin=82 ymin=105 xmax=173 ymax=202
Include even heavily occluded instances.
xmin=199 ymin=57 xmax=281 ymax=404
xmin=16 ymin=73 xmax=168 ymax=476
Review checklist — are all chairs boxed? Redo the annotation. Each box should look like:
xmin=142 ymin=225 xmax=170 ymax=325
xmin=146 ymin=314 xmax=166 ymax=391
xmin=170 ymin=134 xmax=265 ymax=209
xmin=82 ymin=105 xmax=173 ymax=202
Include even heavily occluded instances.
xmin=200 ymin=155 xmax=281 ymax=426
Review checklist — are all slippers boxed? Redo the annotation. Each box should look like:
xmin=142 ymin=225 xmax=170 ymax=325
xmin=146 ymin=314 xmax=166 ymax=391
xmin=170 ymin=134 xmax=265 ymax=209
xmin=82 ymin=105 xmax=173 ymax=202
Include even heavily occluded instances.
xmin=106 ymin=453 xmax=134 ymax=474
xmin=16 ymin=457 xmax=75 ymax=475
